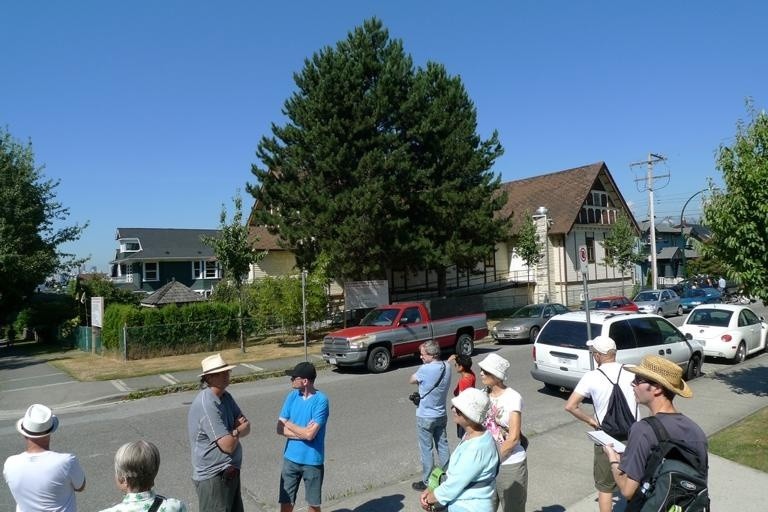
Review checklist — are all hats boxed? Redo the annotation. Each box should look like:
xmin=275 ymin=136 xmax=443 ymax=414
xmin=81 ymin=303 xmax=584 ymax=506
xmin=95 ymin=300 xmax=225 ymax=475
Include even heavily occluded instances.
xmin=285 ymin=361 xmax=317 ymax=380
xmin=451 ymin=385 xmax=491 ymax=425
xmin=197 ymin=353 xmax=237 ymax=376
xmin=16 ymin=404 xmax=59 ymax=438
xmin=478 ymin=352 xmax=510 ymax=381
xmin=586 ymin=336 xmax=617 ymax=354
xmin=622 ymin=356 xmax=693 ymax=398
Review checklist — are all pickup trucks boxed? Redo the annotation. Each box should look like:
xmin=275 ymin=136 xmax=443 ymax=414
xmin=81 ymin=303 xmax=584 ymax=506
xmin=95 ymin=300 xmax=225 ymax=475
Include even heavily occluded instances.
xmin=320 ymin=303 xmax=492 ymax=373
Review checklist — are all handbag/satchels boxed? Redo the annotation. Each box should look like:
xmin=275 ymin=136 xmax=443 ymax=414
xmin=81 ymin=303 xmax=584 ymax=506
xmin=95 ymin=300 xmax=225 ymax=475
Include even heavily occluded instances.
xmin=427 ymin=464 xmax=448 ymax=512
xmin=519 ymin=431 xmax=528 ymax=451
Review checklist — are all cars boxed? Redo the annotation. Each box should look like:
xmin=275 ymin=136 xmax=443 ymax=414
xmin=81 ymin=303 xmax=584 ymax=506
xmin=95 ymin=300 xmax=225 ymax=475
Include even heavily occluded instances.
xmin=576 ymin=277 xmax=734 ymax=320
xmin=673 ymin=302 xmax=768 ymax=365
xmin=489 ymin=301 xmax=576 ymax=344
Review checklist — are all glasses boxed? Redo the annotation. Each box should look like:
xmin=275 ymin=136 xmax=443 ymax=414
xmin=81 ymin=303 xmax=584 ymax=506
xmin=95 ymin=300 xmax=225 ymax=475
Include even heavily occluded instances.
xmin=480 ymin=368 xmax=490 ymax=375
xmin=451 ymin=405 xmax=462 ymax=415
xmin=634 ymin=374 xmax=654 ymax=385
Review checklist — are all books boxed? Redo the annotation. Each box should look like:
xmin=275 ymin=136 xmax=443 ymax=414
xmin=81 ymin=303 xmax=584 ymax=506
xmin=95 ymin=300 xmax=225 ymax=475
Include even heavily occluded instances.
xmin=584 ymin=429 xmax=627 ymax=454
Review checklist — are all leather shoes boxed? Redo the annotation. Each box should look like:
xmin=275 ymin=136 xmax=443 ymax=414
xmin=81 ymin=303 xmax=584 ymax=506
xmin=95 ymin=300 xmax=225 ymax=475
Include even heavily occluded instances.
xmin=412 ymin=480 xmax=427 ymax=490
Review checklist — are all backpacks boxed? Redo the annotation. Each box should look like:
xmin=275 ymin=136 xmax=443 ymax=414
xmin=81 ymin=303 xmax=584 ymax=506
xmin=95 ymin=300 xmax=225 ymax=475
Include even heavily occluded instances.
xmin=593 ymin=363 xmax=638 ymax=442
xmin=624 ymin=416 xmax=710 ymax=512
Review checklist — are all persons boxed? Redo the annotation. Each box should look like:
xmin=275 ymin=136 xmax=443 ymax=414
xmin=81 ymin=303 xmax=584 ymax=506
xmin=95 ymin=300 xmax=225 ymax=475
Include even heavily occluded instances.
xmin=564 ymin=335 xmax=642 ymax=512
xmin=3 ymin=403 xmax=87 ymax=512
xmin=447 ymin=354 xmax=476 ymax=438
xmin=477 ymin=351 xmax=529 ymax=512
xmin=408 ymin=339 xmax=456 ymax=490
xmin=274 ymin=359 xmax=331 ymax=512
xmin=419 ymin=386 xmax=500 ymax=512
xmin=603 ymin=351 xmax=709 ymax=512
xmin=185 ymin=351 xmax=252 ymax=512
xmin=94 ymin=437 xmax=185 ymax=511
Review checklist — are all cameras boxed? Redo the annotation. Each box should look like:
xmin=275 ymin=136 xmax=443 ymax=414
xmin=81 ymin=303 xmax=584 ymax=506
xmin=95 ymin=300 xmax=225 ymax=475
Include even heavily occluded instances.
xmin=409 ymin=392 xmax=420 ymax=405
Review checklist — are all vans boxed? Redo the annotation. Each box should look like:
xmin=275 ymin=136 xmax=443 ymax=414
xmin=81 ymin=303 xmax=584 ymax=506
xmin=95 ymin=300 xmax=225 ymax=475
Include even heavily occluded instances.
xmin=527 ymin=308 xmax=704 ymax=399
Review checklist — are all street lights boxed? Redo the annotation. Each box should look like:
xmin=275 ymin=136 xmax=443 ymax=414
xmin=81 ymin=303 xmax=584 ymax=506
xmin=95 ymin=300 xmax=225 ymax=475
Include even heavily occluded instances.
xmin=299 ymin=266 xmax=310 ymax=360
xmin=680 ymin=186 xmax=720 ymax=275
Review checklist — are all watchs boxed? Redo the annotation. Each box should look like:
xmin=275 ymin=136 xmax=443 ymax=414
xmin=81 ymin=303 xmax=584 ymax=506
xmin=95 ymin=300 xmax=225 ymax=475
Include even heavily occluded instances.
xmin=231 ymin=428 xmax=240 ymax=437
